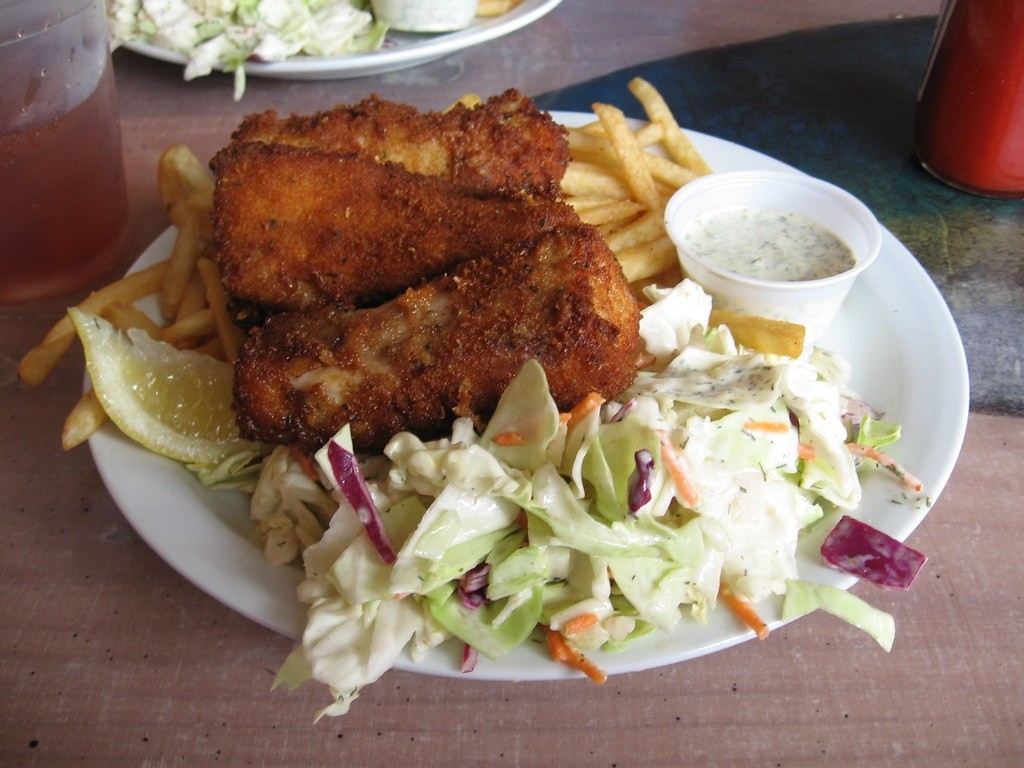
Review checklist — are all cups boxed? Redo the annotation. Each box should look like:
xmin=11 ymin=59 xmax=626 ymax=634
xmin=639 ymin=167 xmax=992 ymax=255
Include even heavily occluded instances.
xmin=0 ymin=0 xmax=132 ymax=307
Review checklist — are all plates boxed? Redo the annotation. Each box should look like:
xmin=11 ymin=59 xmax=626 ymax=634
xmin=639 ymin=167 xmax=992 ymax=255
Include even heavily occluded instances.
xmin=107 ymin=0 xmax=563 ymax=81
xmin=87 ymin=112 xmax=970 ymax=682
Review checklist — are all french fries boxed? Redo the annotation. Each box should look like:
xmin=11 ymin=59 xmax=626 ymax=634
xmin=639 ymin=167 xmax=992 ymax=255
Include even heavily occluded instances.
xmin=16 ymin=77 xmax=713 ymax=453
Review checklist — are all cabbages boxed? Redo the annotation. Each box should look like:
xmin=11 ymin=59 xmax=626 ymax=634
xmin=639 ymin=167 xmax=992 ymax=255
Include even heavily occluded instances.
xmin=101 ymin=0 xmax=389 ymax=100
xmin=168 ymin=272 xmax=897 ymax=725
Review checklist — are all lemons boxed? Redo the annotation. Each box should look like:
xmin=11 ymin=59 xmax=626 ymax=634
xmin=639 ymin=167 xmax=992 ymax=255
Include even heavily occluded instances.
xmin=67 ymin=305 xmax=272 ymax=463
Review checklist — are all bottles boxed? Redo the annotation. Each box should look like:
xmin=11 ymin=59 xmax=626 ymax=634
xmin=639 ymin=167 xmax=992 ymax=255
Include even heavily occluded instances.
xmin=909 ymin=0 xmax=1024 ymax=203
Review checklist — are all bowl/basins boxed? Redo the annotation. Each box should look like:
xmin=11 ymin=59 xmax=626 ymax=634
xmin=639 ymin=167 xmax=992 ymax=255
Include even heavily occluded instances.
xmin=666 ymin=170 xmax=883 ymax=348
